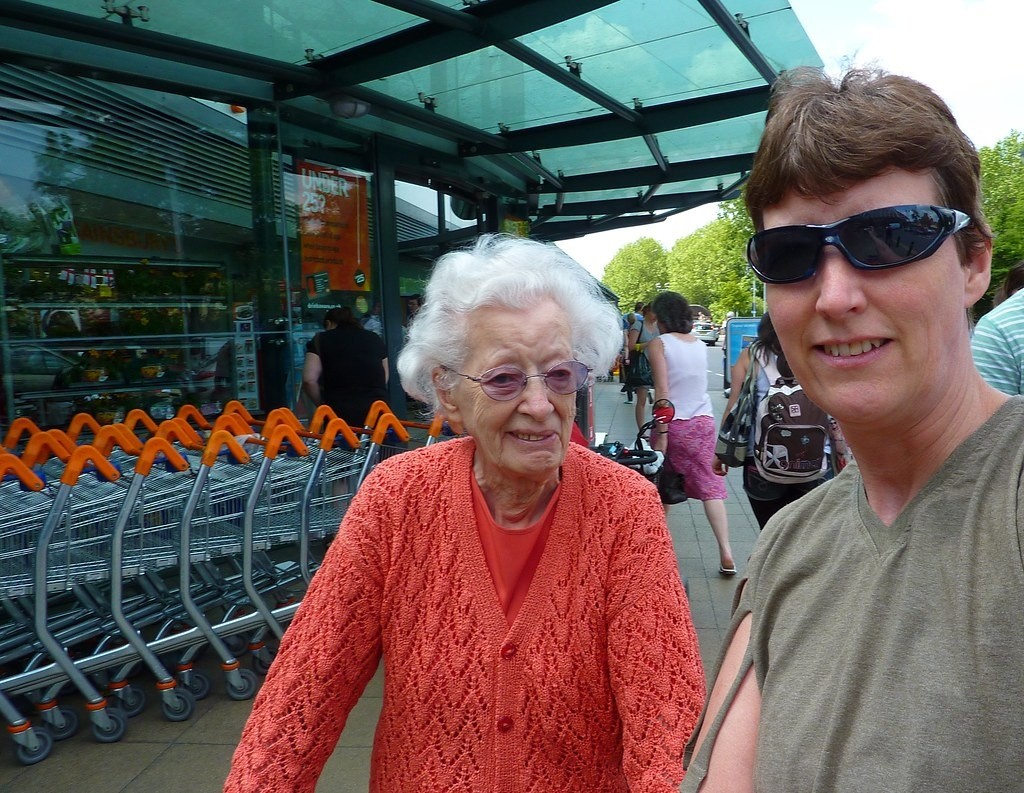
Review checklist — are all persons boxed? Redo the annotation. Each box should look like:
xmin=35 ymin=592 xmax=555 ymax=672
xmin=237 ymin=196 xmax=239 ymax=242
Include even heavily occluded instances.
xmin=221 ymin=232 xmax=736 ymax=793
xmin=685 ymin=61 xmax=1024 ymax=793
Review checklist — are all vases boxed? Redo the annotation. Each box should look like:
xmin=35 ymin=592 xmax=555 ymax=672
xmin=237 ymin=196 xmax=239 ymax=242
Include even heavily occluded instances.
xmin=201 ymin=403 xmax=223 ymax=415
xmin=96 ymin=410 xmax=120 ymax=425
xmin=150 ymin=402 xmax=176 ymax=420
xmin=141 ymin=366 xmax=161 ymax=378
xmin=83 ymin=370 xmax=109 ymax=382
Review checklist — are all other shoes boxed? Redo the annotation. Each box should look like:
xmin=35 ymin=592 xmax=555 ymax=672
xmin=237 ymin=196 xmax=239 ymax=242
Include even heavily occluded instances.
xmin=414 ymin=408 xmax=435 ymax=421
xmin=621 ymin=385 xmax=626 ymax=392
xmin=624 ymin=400 xmax=633 ymax=404
xmin=648 ymin=392 xmax=653 ymax=404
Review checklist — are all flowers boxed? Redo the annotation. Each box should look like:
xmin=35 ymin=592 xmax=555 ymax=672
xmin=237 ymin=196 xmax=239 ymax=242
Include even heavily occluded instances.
xmin=8 ymin=259 xmax=224 ymax=425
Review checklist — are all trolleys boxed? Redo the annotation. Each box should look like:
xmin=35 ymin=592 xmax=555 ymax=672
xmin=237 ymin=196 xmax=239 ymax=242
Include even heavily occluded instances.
xmin=0 ymin=401 xmax=470 ymax=767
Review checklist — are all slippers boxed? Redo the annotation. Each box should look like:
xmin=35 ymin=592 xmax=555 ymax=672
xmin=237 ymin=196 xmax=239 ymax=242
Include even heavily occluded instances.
xmin=719 ymin=563 xmax=737 ymax=574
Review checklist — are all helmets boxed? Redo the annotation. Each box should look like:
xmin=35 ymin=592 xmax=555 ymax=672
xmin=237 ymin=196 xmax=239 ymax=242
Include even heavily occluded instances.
xmin=10 ymin=265 xmax=224 ymax=426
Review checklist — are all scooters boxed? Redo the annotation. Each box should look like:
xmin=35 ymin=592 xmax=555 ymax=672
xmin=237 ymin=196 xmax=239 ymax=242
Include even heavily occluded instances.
xmin=584 ymin=398 xmax=677 ymax=473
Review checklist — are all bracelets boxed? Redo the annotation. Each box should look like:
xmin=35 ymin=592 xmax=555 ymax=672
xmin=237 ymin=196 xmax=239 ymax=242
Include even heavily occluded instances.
xmin=654 ymin=429 xmax=669 ymax=435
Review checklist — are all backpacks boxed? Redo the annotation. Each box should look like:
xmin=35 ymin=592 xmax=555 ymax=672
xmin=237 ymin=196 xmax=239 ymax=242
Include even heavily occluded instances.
xmin=753 ymin=376 xmax=831 ymax=484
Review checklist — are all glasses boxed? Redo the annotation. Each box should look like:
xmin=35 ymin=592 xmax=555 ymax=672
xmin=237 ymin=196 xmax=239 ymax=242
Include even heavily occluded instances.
xmin=440 ymin=360 xmax=594 ymax=401
xmin=408 ymin=302 xmax=418 ymax=307
xmin=747 ymin=204 xmax=972 ymax=284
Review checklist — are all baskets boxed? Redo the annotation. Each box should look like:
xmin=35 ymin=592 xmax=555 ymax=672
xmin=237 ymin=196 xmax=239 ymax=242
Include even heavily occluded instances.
xmin=645 ymin=464 xmax=665 ymax=488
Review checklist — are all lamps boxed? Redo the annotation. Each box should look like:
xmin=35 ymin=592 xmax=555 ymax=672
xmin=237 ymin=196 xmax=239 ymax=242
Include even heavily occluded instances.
xmin=330 ymin=95 xmax=372 ymax=119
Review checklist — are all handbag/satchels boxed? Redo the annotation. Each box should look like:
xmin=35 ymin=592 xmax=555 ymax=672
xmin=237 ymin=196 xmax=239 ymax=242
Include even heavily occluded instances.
xmin=625 ymin=350 xmax=655 ymax=391
xmin=658 ymin=451 xmax=687 ymax=504
xmin=714 ymin=344 xmax=758 ymax=468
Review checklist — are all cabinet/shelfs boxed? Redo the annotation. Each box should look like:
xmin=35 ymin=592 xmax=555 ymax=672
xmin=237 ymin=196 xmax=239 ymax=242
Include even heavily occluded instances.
xmin=0 ymin=253 xmax=238 ymax=443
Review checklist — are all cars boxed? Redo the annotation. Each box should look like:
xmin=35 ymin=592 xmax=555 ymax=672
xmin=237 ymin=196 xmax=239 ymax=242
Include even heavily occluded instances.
xmin=691 ymin=316 xmax=720 ymax=345
